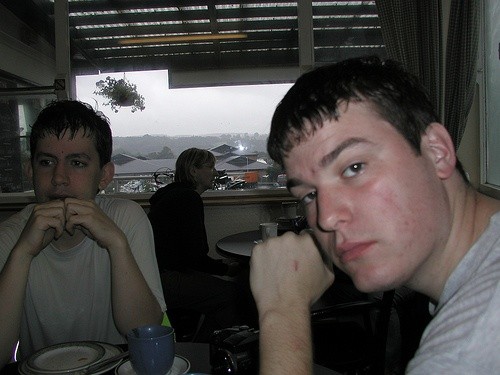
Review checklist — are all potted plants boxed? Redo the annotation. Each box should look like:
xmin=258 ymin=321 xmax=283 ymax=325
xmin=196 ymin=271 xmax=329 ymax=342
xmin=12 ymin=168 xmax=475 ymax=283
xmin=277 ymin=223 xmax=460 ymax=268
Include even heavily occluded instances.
xmin=92 ymin=74 xmax=146 ymax=113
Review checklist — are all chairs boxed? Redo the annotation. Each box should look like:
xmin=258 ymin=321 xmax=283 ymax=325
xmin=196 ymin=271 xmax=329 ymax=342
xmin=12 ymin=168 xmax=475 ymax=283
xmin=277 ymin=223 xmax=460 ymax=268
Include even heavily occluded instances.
xmin=311 ymin=287 xmax=395 ymax=375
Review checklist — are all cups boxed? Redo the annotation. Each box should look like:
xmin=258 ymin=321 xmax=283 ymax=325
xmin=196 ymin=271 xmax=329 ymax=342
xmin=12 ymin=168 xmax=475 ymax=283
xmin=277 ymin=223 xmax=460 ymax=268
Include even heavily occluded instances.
xmin=127 ymin=325 xmax=175 ymax=375
xmin=282 ymin=202 xmax=297 ymax=217
xmin=260 ymin=223 xmax=281 ymax=242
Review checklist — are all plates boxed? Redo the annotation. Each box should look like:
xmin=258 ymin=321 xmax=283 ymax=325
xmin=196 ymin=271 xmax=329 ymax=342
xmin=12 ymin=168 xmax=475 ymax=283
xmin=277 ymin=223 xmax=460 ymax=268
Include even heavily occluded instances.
xmin=116 ymin=356 xmax=191 ymax=374
xmin=19 ymin=343 xmax=125 ymax=375
xmin=25 ymin=340 xmax=106 ymax=373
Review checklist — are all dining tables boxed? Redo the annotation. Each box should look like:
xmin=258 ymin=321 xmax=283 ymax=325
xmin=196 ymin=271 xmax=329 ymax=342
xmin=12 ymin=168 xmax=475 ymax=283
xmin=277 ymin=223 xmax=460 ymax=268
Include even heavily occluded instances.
xmin=0 ymin=342 xmax=341 ymax=374
xmin=215 ymin=228 xmax=301 ymax=263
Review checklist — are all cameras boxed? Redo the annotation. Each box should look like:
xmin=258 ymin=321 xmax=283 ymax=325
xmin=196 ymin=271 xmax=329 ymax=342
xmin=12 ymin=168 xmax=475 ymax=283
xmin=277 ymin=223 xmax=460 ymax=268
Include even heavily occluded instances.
xmin=209 ymin=324 xmax=258 ymax=375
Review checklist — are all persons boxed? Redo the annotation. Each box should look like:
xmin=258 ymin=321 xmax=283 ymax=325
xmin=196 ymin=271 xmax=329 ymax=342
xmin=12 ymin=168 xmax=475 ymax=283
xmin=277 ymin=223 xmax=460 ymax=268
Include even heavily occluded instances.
xmin=250 ymin=54 xmax=500 ymax=375
xmin=147 ymin=148 xmax=241 ymax=329
xmin=0 ymin=100 xmax=172 ymax=369
